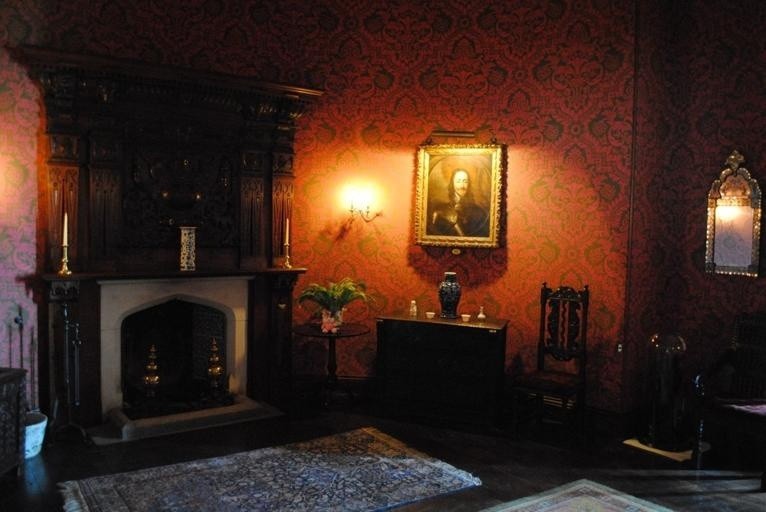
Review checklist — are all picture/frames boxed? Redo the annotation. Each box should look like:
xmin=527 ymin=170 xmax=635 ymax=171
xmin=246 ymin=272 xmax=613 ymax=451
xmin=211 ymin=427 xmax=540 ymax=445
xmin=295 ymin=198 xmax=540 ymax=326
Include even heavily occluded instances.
xmin=702 ymin=145 xmax=764 ymax=282
xmin=410 ymin=142 xmax=506 ymax=255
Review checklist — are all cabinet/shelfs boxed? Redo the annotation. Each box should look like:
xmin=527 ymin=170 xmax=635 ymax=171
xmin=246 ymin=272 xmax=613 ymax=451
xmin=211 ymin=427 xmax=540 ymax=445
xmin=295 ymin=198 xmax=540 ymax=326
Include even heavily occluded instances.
xmin=373 ymin=308 xmax=511 ymax=433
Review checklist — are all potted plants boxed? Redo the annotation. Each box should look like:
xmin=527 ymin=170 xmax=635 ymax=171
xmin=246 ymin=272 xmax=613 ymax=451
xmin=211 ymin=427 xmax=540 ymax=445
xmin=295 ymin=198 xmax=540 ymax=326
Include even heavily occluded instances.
xmin=293 ymin=277 xmax=375 ymax=333
xmin=22 ymin=410 xmax=50 ymax=461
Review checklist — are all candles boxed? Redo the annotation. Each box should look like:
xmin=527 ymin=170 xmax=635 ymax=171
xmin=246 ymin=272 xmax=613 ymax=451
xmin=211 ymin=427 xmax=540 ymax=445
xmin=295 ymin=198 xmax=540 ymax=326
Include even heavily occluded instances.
xmin=60 ymin=211 xmax=69 ymax=247
xmin=284 ymin=217 xmax=290 ymax=245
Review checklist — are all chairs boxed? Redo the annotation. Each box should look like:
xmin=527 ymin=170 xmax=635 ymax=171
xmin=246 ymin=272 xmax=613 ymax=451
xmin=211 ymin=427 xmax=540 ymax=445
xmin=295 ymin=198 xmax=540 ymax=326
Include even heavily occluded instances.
xmin=692 ymin=310 xmax=764 ymax=495
xmin=506 ymin=278 xmax=594 ymax=469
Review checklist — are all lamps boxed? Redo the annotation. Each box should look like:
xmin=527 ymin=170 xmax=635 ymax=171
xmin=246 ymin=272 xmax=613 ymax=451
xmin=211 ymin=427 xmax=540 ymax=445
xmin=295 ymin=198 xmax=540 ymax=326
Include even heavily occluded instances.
xmin=343 ymin=199 xmax=383 ymax=225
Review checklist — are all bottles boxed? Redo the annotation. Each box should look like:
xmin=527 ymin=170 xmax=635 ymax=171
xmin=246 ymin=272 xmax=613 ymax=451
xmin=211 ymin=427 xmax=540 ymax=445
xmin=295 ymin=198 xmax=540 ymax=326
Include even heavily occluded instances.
xmin=409 ymin=299 xmax=418 ymax=317
xmin=477 ymin=306 xmax=486 ymax=321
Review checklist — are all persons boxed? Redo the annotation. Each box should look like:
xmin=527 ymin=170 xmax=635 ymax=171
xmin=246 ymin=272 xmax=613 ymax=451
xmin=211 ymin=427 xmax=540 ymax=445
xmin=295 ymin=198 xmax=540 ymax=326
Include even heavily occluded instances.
xmin=427 ymin=164 xmax=492 ymax=239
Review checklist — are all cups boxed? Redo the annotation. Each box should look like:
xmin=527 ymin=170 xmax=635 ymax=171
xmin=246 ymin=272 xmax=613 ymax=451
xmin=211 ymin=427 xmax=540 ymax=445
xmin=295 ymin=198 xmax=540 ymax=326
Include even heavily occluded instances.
xmin=426 ymin=312 xmax=436 ymax=319
xmin=462 ymin=314 xmax=471 ymax=322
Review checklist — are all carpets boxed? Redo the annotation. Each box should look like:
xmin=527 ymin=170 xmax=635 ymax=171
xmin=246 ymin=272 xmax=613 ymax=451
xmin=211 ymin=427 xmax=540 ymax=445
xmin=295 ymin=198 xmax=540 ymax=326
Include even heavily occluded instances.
xmin=53 ymin=424 xmax=482 ymax=512
xmin=471 ymin=474 xmax=685 ymax=512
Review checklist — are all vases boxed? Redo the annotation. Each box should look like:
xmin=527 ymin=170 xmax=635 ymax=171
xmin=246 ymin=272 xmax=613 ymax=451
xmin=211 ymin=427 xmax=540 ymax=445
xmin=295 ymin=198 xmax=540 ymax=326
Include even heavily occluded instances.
xmin=178 ymin=226 xmax=198 ymax=272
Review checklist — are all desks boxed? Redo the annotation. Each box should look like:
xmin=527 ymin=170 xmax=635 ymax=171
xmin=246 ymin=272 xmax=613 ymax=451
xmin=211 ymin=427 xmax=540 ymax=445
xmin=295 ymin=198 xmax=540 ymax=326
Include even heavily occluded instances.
xmin=290 ymin=320 xmax=371 ymax=411
